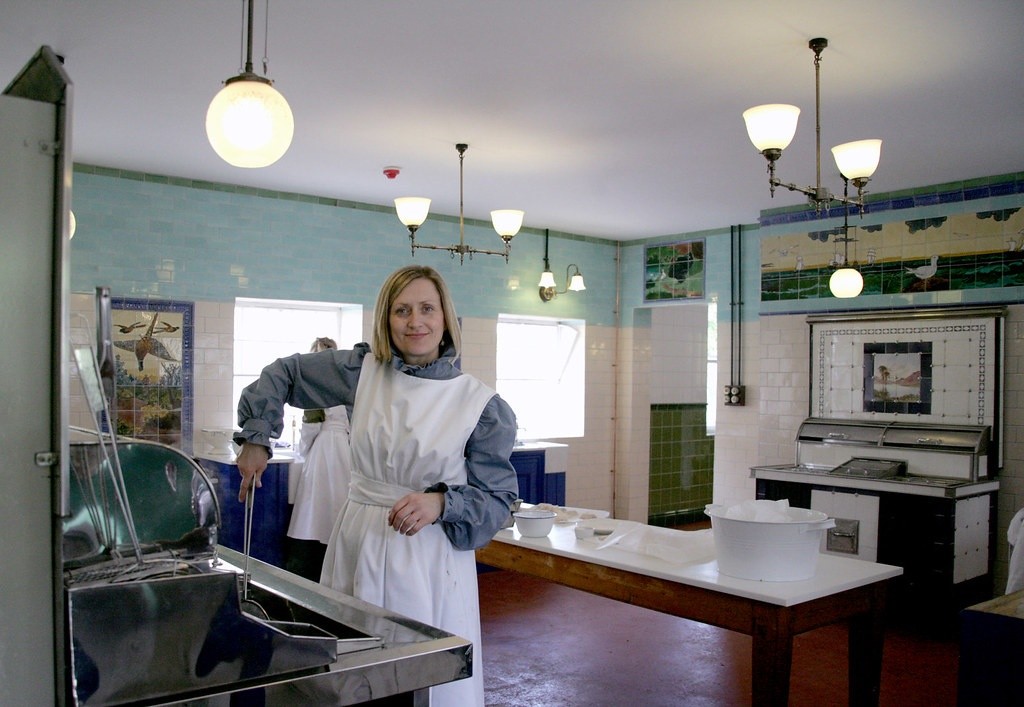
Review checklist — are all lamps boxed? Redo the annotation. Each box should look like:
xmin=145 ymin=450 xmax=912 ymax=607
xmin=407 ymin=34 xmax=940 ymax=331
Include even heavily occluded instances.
xmin=539 ymin=228 xmax=586 ymax=301
xmin=204 ymin=0 xmax=296 ymax=168
xmin=394 ymin=144 xmax=522 ymax=265
xmin=743 ymin=38 xmax=884 ymax=299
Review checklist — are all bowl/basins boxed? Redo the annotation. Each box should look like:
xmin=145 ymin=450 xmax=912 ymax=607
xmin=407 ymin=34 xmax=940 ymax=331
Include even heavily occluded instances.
xmin=574 ymin=526 xmax=595 ymax=538
xmin=512 ymin=510 xmax=558 ymax=538
xmin=704 ymin=500 xmax=836 ymax=584
xmin=500 ymin=499 xmax=524 ymax=529
xmin=201 ymin=428 xmax=276 ymax=457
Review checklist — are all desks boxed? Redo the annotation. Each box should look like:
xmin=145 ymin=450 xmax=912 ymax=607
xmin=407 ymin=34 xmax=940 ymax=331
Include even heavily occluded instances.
xmin=955 ymin=589 xmax=1024 ymax=707
xmin=478 ymin=502 xmax=904 ymax=706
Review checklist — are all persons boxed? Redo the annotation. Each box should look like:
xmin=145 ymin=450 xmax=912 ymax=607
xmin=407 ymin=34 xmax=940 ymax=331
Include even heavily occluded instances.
xmin=287 ymin=337 xmax=355 ymax=584
xmin=232 ymin=265 xmax=519 ymax=707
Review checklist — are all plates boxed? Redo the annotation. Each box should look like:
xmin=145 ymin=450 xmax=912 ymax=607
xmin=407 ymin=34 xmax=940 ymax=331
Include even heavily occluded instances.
xmin=530 ymin=503 xmax=610 ymax=523
xmin=575 ymin=518 xmax=642 ymax=544
